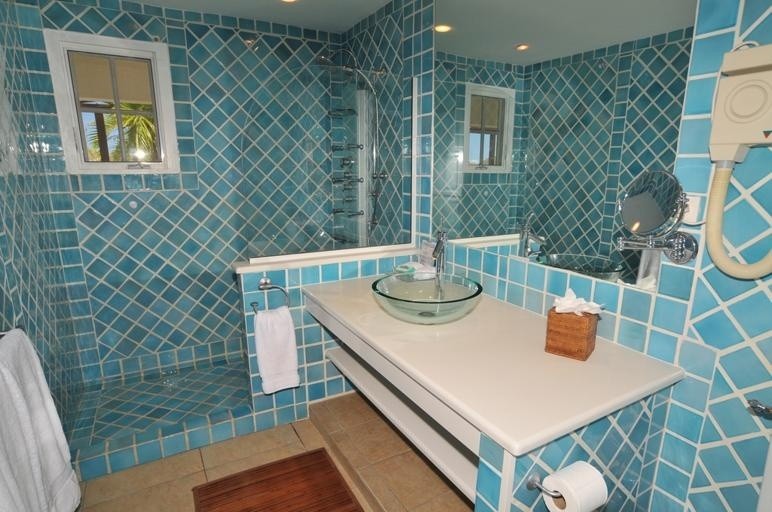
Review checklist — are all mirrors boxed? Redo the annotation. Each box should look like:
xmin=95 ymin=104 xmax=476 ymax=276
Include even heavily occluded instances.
xmin=428 ymin=0 xmax=702 ymax=297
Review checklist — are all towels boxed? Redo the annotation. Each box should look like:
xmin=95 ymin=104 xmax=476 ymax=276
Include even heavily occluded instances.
xmin=0 ymin=327 xmax=84 ymax=511
xmin=253 ymin=305 xmax=300 ymax=395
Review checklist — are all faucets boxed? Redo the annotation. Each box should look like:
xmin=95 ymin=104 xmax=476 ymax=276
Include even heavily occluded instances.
xmin=518 ymin=214 xmax=547 ymax=257
xmin=432 ymin=231 xmax=447 ymax=272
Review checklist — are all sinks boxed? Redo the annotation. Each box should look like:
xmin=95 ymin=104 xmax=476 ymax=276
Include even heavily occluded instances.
xmin=371 ymin=272 xmax=483 ymax=326
xmin=538 ymin=255 xmax=624 ymax=282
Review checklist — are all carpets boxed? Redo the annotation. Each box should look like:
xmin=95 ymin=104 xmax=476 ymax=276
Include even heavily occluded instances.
xmin=191 ymin=446 xmax=364 ymax=512
